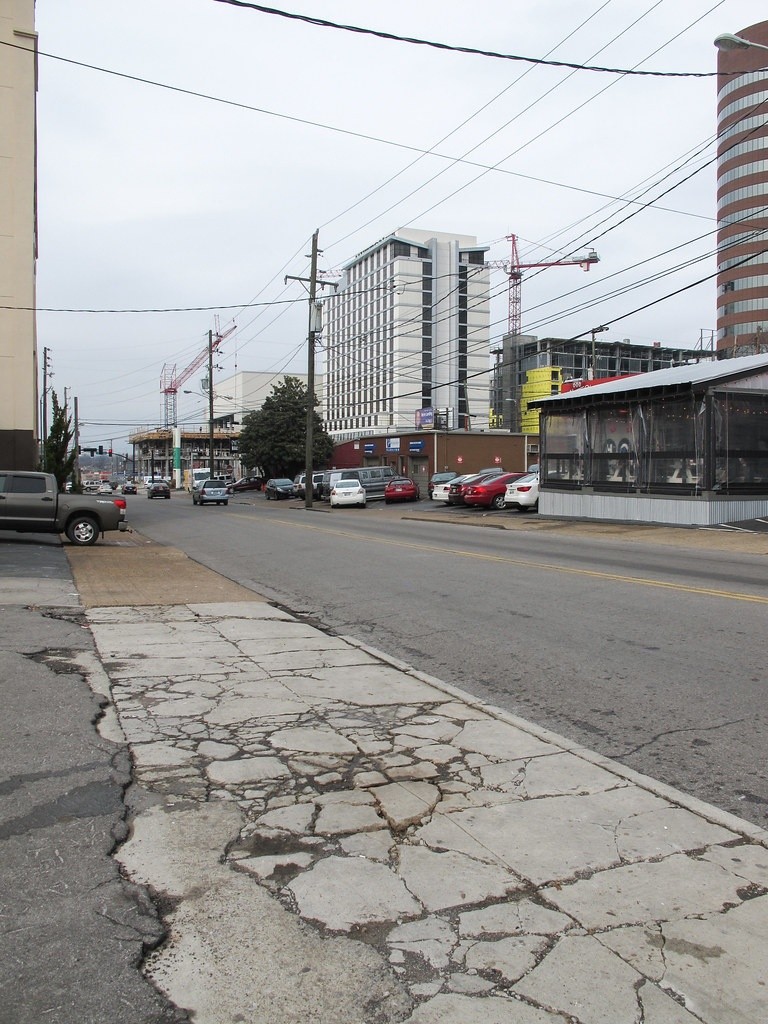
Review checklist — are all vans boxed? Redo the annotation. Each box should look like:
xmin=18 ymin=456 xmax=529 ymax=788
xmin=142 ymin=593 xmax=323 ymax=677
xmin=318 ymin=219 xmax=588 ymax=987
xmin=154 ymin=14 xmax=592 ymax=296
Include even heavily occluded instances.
xmin=214 ymin=474 xmax=234 ymax=485
xmin=321 ymin=466 xmax=399 ymax=500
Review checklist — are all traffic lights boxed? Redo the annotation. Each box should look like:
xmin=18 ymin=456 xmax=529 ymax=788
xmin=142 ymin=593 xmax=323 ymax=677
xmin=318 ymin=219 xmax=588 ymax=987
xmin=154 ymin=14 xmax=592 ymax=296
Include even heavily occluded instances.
xmin=108 ymin=448 xmax=113 ymax=458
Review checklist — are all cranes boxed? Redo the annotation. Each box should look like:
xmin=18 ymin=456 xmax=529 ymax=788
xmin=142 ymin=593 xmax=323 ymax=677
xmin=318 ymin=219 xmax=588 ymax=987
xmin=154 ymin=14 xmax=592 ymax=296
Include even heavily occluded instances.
xmin=159 ymin=314 xmax=239 ymax=432
xmin=471 ymin=233 xmax=601 ymax=335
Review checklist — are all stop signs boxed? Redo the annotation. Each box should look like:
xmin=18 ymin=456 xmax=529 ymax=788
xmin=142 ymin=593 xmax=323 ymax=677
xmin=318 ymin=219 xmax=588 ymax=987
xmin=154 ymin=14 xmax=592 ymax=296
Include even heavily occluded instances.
xmin=225 ymin=465 xmax=233 ymax=474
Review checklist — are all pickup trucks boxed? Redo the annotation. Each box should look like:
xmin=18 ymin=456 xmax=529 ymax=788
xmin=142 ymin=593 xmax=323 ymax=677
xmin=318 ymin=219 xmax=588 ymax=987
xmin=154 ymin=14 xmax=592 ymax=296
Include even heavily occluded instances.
xmin=0 ymin=470 xmax=132 ymax=546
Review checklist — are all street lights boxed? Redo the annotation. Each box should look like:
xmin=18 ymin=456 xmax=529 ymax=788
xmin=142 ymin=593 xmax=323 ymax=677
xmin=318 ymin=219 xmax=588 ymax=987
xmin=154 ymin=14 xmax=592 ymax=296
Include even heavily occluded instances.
xmin=591 ymin=327 xmax=609 ymax=380
xmin=184 ymin=390 xmax=233 ymax=481
xmin=64 ymin=423 xmax=84 ymax=464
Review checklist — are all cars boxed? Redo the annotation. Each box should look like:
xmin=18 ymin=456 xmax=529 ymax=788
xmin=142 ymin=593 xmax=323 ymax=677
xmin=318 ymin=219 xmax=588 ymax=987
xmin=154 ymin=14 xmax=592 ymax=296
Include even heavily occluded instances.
xmin=385 ymin=477 xmax=419 ymax=504
xmin=144 ymin=478 xmax=171 ymax=500
xmin=226 ymin=476 xmax=263 ymax=493
xmin=66 ymin=478 xmax=117 ymax=495
xmin=427 ymin=464 xmax=567 ymax=512
xmin=122 ymin=483 xmax=138 ymax=495
xmin=330 ymin=479 xmax=367 ymax=508
xmin=193 ymin=480 xmax=229 ymax=506
xmin=265 ymin=478 xmax=299 ymax=500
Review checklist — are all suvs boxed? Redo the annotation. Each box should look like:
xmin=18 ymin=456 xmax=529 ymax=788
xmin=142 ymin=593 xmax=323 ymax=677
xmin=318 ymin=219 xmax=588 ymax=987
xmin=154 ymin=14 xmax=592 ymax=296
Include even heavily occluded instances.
xmin=293 ymin=472 xmax=324 ymax=500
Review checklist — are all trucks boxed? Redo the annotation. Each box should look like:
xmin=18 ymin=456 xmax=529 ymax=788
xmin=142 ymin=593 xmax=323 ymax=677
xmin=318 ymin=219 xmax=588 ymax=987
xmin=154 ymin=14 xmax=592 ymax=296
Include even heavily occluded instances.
xmin=184 ymin=468 xmax=210 ymax=494
